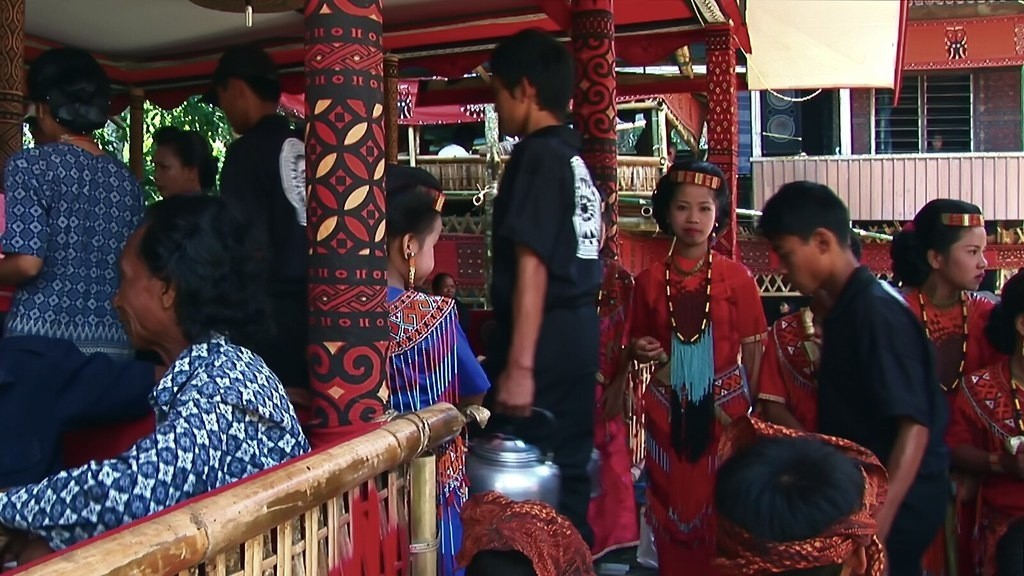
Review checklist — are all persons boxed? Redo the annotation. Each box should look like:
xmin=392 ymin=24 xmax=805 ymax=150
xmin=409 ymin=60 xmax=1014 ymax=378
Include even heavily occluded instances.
xmin=0 ymin=25 xmax=1024 ymax=576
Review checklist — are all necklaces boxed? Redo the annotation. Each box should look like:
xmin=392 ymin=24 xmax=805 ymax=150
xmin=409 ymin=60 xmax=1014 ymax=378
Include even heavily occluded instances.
xmin=59 ymin=134 xmax=93 ymax=143
xmin=1008 ymin=357 xmax=1024 ymax=435
xmin=671 ymin=254 xmax=706 ymax=279
xmin=917 ymin=287 xmax=968 ymax=393
xmin=664 ymin=245 xmax=716 ymax=464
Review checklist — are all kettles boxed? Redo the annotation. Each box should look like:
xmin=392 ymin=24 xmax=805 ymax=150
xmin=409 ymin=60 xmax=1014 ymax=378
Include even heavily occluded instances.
xmin=463 ymin=407 xmax=561 ymax=516
